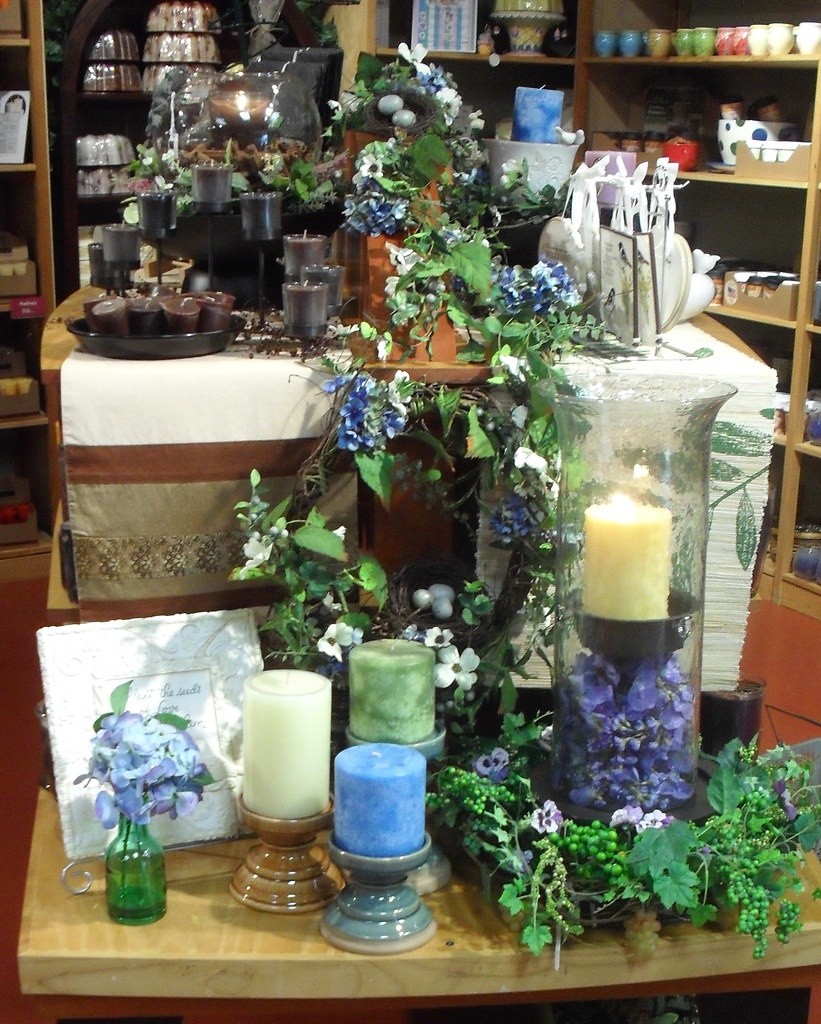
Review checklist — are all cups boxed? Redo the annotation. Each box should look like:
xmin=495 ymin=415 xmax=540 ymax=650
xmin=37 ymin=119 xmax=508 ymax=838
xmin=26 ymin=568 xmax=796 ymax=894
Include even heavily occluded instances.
xmin=592 ymin=20 xmax=821 ymax=57
xmin=719 ymin=93 xmax=780 ymax=120
xmin=605 ymin=129 xmax=662 ymax=156
xmin=708 ymin=259 xmax=790 ymax=307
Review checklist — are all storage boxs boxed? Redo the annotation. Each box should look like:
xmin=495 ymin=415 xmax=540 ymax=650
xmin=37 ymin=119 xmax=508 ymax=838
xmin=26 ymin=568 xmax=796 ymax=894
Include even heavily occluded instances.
xmin=0 ymin=231 xmax=36 ymax=298
xmin=0 ymin=475 xmax=39 ymax=546
xmin=0 ymin=0 xmax=23 ymax=39
xmin=721 ymin=270 xmax=800 ymax=321
xmin=0 ymin=351 xmax=41 ymax=417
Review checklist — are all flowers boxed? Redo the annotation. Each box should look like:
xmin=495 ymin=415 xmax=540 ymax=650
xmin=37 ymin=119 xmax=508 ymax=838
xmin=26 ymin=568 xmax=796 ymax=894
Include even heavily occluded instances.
xmin=461 ymin=652 xmax=795 ymax=862
xmin=221 ymin=41 xmax=584 ymax=705
xmin=71 ymin=678 xmax=219 ymax=894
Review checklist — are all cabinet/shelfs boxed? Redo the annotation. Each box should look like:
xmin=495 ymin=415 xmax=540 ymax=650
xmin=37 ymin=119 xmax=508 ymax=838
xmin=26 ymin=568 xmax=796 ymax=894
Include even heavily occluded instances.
xmin=573 ymin=0 xmax=821 ymax=625
xmin=62 ymin=0 xmax=336 ymax=315
xmin=0 ymin=0 xmax=60 ymax=585
xmin=323 ymin=0 xmax=578 ymax=145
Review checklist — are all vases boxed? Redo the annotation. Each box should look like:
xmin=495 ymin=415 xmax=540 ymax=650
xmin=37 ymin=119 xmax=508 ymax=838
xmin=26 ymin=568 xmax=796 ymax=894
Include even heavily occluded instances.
xmin=105 ymin=791 xmax=167 ymax=926
xmin=532 ymin=369 xmax=742 ymax=814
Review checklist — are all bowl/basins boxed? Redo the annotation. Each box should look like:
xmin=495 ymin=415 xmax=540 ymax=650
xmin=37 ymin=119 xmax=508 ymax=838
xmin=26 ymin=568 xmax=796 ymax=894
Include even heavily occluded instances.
xmin=717 ymin=120 xmax=803 ymax=165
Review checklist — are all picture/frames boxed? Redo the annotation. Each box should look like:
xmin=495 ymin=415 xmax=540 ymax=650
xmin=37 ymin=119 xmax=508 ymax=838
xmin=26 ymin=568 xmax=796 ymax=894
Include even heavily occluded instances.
xmin=598 ymin=224 xmax=662 ymax=349
xmin=409 ymin=0 xmax=479 ymax=54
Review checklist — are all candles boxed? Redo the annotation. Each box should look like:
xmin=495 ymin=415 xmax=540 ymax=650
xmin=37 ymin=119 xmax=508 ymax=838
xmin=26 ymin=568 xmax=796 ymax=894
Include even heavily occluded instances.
xmin=584 ymin=149 xmax=636 ymax=205
xmin=0 ymin=259 xmax=29 ymax=277
xmin=580 ymin=485 xmax=674 ymax=622
xmin=0 ymin=376 xmax=33 ymax=397
xmin=75 ymin=72 xmax=349 ymax=343
xmin=241 ymin=669 xmax=332 ymax=819
xmin=348 ymin=638 xmax=436 ymax=744
xmin=331 ymin=742 xmax=428 ymax=858
xmin=0 ymin=501 xmax=34 ymax=525
xmin=510 ymin=83 xmax=566 ymax=145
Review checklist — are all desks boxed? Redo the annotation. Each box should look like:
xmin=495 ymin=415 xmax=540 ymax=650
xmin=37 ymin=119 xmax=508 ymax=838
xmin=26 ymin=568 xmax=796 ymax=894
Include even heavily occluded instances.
xmin=15 ymin=777 xmax=821 ymax=1024
